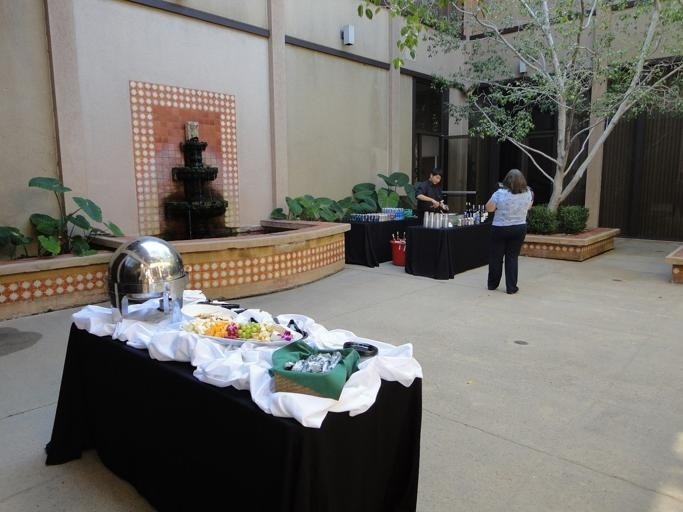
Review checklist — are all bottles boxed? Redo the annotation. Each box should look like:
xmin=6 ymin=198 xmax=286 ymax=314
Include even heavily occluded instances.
xmin=464 ymin=202 xmax=485 ymax=226
xmin=381 ymin=208 xmax=404 ymax=221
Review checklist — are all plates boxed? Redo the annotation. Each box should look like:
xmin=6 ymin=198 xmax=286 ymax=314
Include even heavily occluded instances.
xmin=198 ymin=321 xmax=303 ymax=347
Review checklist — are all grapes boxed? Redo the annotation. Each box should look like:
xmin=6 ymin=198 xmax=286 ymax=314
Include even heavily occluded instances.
xmin=236 ymin=321 xmax=260 ymax=339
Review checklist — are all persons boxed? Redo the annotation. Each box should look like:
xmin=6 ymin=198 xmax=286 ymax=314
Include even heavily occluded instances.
xmin=485 ymin=169 xmax=534 ymax=294
xmin=414 ymin=168 xmax=449 ymax=225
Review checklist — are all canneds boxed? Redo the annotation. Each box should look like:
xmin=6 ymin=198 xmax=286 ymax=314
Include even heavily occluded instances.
xmin=350 ymin=207 xmax=405 ymax=222
xmin=458 ymin=217 xmax=474 ymax=226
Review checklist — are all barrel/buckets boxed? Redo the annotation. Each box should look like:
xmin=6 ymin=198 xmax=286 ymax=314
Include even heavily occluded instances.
xmin=390 ymin=239 xmax=406 ymax=266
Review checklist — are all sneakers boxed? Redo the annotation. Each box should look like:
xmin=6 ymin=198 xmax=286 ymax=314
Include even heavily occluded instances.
xmin=507 ymin=287 xmax=518 ymax=294
